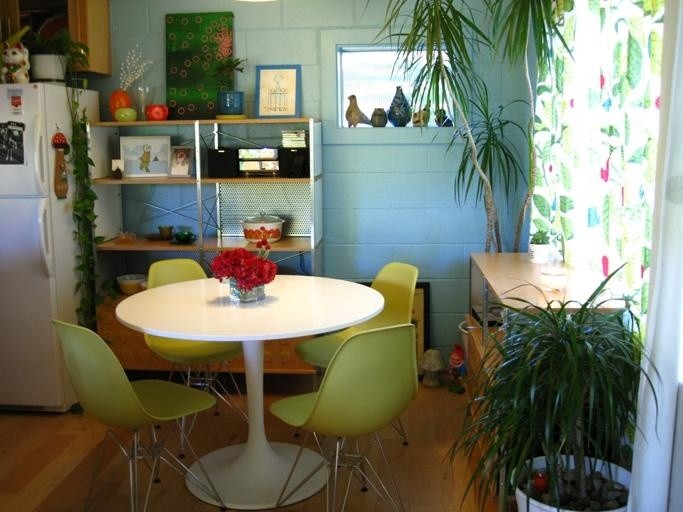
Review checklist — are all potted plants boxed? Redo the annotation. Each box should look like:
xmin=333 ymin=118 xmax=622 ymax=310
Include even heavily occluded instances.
xmin=359 ymin=0 xmax=575 ymax=364
xmin=212 ymin=56 xmax=248 ymax=115
xmin=30 ymin=29 xmax=121 ymax=346
xmin=449 ymin=261 xmax=662 ymax=512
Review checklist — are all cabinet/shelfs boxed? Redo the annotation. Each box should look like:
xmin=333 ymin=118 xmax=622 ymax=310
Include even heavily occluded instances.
xmin=84 ymin=117 xmax=324 ymax=379
xmin=458 ymin=251 xmax=627 ymax=500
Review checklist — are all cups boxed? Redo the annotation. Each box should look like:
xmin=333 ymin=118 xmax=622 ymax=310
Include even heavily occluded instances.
xmin=177 ymin=231 xmax=197 ymax=243
xmin=158 ymin=225 xmax=175 ymax=235
xmin=541 ymin=265 xmax=569 ymax=291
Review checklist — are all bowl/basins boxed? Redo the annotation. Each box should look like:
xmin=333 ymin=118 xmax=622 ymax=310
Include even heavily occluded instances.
xmin=116 ymin=273 xmax=147 ymax=294
xmin=140 ymin=280 xmax=148 ymax=291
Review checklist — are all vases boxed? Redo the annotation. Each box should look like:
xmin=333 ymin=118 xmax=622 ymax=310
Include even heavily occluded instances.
xmin=227 ymin=279 xmax=265 ymax=303
xmin=108 ymin=88 xmax=129 ymax=121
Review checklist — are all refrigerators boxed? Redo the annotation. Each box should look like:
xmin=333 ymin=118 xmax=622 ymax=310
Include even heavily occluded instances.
xmin=0 ymin=83 xmax=101 ymax=415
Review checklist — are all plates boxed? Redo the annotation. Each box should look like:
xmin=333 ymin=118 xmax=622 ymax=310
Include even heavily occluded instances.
xmin=145 ymin=235 xmax=173 ymax=241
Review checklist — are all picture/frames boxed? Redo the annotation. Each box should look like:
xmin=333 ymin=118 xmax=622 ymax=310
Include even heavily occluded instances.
xmin=170 ymin=144 xmax=194 ymax=178
xmin=255 ymin=65 xmax=301 ymax=118
xmin=358 ymin=280 xmax=430 ymax=383
xmin=120 ymin=134 xmax=172 ymax=178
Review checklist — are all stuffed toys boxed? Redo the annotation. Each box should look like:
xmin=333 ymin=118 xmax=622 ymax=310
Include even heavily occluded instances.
xmin=447 ymin=344 xmax=468 ymax=395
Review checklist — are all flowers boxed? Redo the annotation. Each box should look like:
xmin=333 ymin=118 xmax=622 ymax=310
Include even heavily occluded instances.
xmin=209 ymin=238 xmax=276 ymax=295
xmin=106 ymin=43 xmax=153 ymax=91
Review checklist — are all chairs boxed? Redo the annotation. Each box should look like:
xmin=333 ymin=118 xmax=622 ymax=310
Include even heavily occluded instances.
xmin=269 ymin=323 xmax=417 ymax=512
xmin=144 ymin=257 xmax=248 ymax=460
xmin=52 ymin=319 xmax=228 ymax=512
xmin=293 ymin=262 xmax=417 ymax=493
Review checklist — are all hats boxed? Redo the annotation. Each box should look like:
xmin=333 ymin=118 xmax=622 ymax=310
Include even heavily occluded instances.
xmin=453 ymin=345 xmax=463 ymax=355
